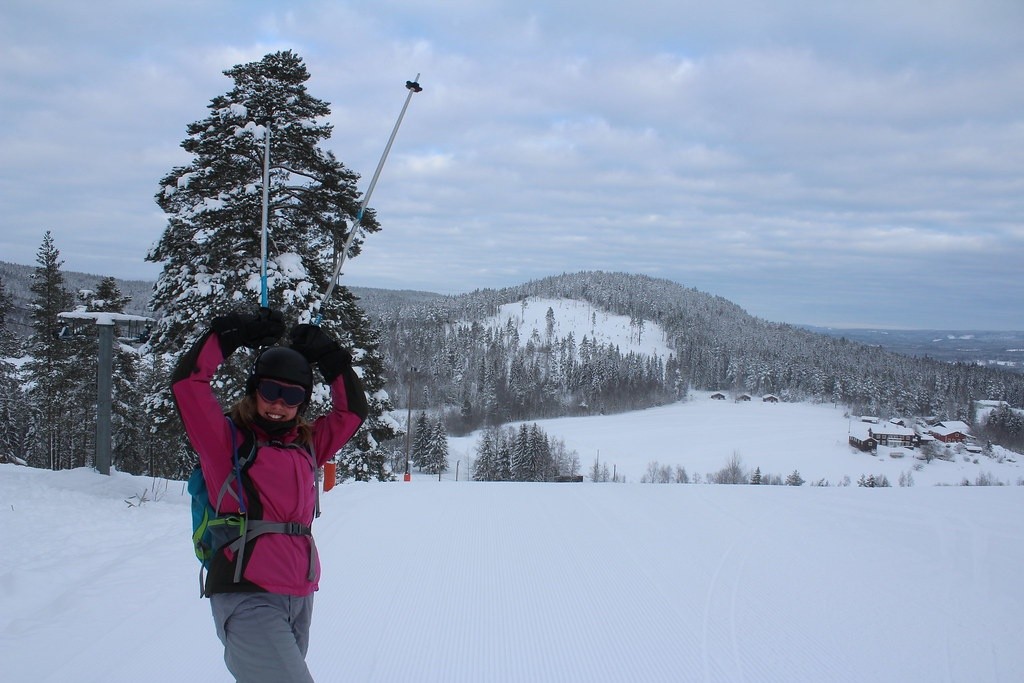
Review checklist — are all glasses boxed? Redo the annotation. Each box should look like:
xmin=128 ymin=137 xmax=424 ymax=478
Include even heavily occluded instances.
xmin=256 ymin=379 xmax=305 ymax=407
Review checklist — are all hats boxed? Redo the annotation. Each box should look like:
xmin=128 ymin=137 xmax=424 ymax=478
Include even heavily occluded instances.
xmin=245 ymin=347 xmax=312 ymax=412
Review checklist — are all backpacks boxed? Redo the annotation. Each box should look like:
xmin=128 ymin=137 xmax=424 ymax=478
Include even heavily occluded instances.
xmin=187 ymin=423 xmax=321 ymax=583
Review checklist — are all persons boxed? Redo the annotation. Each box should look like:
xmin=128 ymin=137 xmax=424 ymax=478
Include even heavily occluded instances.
xmin=170 ymin=308 xmax=368 ymax=683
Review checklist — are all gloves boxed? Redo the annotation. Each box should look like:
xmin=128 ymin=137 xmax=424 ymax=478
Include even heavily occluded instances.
xmin=289 ymin=324 xmax=348 ymax=378
xmin=212 ymin=307 xmax=285 ymax=358
